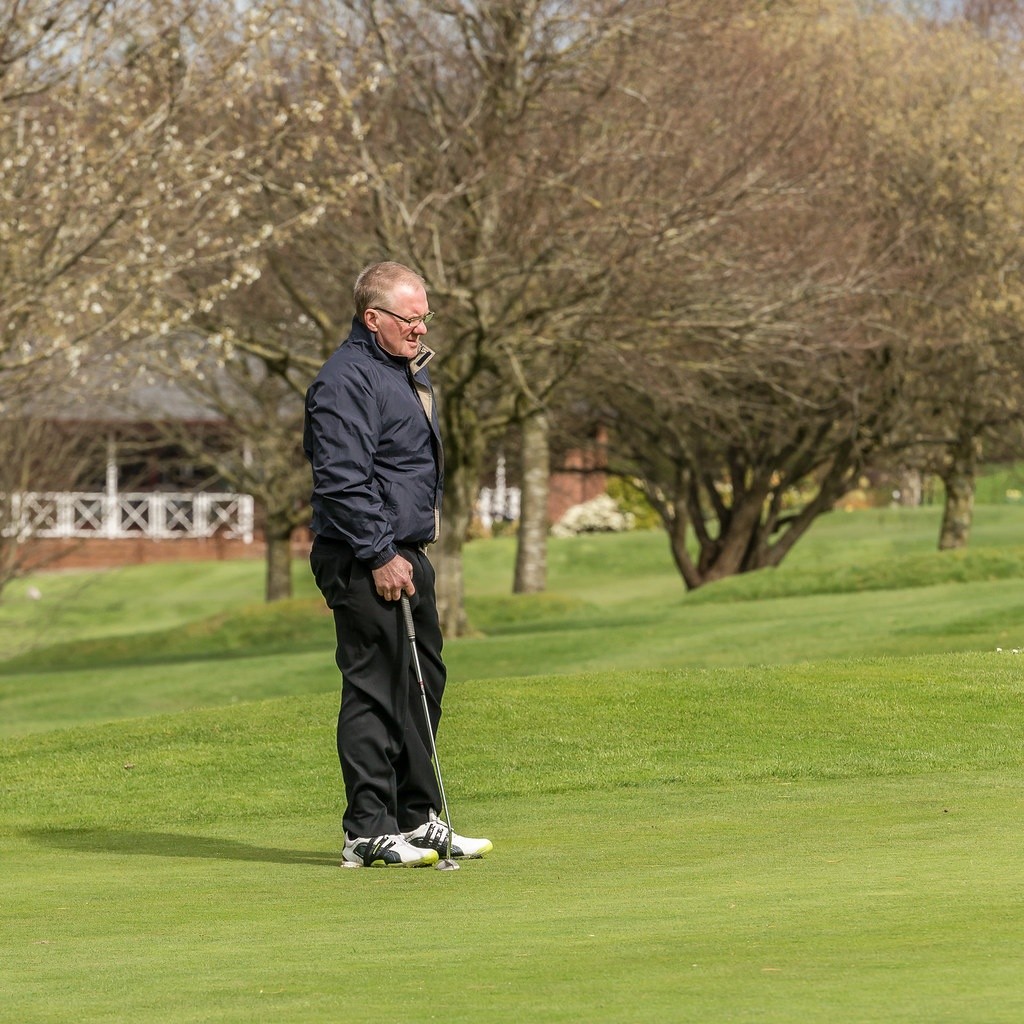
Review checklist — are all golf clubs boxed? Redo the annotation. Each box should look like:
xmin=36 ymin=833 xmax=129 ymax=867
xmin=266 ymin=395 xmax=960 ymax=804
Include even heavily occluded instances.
xmin=400 ymin=587 xmax=461 ymax=871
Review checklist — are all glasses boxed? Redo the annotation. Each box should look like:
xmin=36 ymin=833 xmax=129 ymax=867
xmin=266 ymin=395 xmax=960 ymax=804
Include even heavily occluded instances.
xmin=375 ymin=305 xmax=434 ymax=328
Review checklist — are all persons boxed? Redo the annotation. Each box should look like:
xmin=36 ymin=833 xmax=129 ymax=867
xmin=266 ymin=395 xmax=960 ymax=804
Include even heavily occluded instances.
xmin=301 ymin=261 xmax=493 ymax=870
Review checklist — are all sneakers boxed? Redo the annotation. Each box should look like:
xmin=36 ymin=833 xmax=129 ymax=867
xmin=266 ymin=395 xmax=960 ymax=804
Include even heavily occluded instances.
xmin=400 ymin=807 xmax=494 ymax=859
xmin=340 ymin=831 xmax=439 ymax=870
